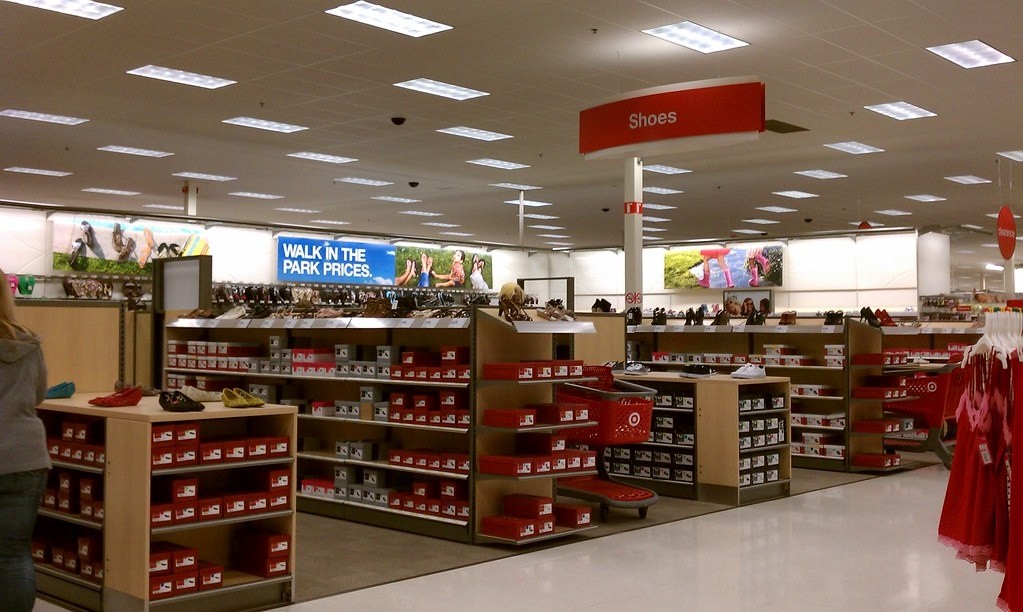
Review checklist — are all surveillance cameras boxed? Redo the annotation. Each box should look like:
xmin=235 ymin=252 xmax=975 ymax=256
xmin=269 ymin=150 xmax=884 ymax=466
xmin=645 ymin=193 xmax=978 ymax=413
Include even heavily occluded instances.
xmin=391 ymin=118 xmax=406 ymax=125
xmin=805 ymin=219 xmax=812 ymax=223
xmin=409 ymin=182 xmax=419 ymax=187
xmin=602 ymin=209 xmax=609 ymax=212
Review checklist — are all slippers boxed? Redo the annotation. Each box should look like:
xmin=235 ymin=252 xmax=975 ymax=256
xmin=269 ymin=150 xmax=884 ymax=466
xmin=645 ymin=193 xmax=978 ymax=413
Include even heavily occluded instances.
xmin=69 ymin=239 xmax=83 ymax=266
xmin=81 ymin=221 xmax=93 ymax=247
xmin=117 ymin=238 xmax=134 ymax=263
xmin=538 ymin=303 xmax=576 ymax=322
xmin=8 ymin=275 xmax=35 ymax=296
xmin=112 ymin=223 xmax=122 ymax=251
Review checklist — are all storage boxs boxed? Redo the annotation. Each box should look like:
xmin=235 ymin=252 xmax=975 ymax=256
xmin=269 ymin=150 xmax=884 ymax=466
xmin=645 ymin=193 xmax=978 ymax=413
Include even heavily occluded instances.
xmin=42 ymin=485 xmax=58 ymax=510
xmin=94 ymin=444 xmax=105 ymax=468
xmin=59 ymin=471 xmax=81 ymax=494
xmin=48 ymin=439 xmax=62 ymax=459
xmin=34 ymin=540 xmax=56 ymax=564
xmin=57 ymin=492 xmax=80 ymax=514
xmin=61 ymin=420 xmax=74 ymax=442
xmin=71 ymin=444 xmax=84 ymax=464
xmin=77 ymin=536 xmax=99 ymax=562
xmin=51 ymin=544 xmax=72 ymax=569
xmin=79 ymin=478 xmax=100 ymax=500
xmin=149 ymin=334 xmax=971 ymax=602
xmin=93 ymin=501 xmax=104 ymax=523
xmin=95 ymin=560 xmax=102 ymax=584
xmin=59 ymin=442 xmax=73 ymax=463
xmin=82 ymin=445 xmax=96 ymax=467
xmin=79 ymin=500 xmax=94 ymax=520
xmin=74 ymin=422 xmax=97 ymax=443
xmin=79 ymin=560 xmax=98 ymax=581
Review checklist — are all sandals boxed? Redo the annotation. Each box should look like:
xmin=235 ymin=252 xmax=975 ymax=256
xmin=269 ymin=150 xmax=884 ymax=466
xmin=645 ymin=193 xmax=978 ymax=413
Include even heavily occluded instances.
xmin=122 ymin=280 xmax=144 ymax=297
xmin=138 ymin=247 xmax=151 ymax=268
xmin=144 ymin=229 xmax=154 ymax=248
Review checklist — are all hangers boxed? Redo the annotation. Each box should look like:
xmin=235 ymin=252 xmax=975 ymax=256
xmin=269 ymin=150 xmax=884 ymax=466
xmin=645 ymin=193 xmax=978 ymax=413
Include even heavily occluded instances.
xmin=961 ymin=311 xmax=1023 ymax=369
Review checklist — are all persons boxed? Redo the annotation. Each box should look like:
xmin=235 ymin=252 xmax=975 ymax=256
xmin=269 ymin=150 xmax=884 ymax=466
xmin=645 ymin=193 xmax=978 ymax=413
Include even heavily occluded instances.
xmin=394 ymin=258 xmax=416 ymax=286
xmin=417 ymin=254 xmax=433 ymax=287
xmin=726 ymin=297 xmax=769 ymax=316
xmin=697 ymin=248 xmax=734 ymax=288
xmin=469 ymin=254 xmax=489 ymax=289
xmin=431 ymin=250 xmax=466 ymax=288
xmin=0 ymin=269 xmax=53 ymax=612
xmin=748 ymin=247 xmax=771 ymax=286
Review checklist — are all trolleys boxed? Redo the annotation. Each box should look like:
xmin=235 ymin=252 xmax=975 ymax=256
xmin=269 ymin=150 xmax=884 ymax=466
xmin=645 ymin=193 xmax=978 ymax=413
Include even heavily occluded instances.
xmin=553 ymin=364 xmax=660 ymax=522
xmin=883 ymin=357 xmax=972 ymax=473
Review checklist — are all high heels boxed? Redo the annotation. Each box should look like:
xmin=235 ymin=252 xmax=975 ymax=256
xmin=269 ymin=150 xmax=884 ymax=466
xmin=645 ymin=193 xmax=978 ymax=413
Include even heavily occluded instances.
xmin=746 ymin=310 xmax=767 ymax=325
xmin=684 ymin=307 xmax=704 ymax=325
xmin=860 ymin=307 xmax=880 ymax=327
xmin=875 ymin=309 xmax=898 ymax=327
xmin=711 ymin=309 xmax=730 ymax=325
xmin=498 ymin=294 xmax=533 ymax=322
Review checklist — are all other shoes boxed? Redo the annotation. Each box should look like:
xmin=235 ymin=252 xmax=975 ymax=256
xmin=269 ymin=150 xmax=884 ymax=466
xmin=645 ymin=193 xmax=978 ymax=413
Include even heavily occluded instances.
xmin=88 ymin=386 xmax=142 ymax=407
xmin=651 ymin=312 xmax=667 ymax=325
xmin=591 ymin=298 xmax=611 ymax=312
xmin=63 ymin=278 xmax=113 ymax=299
xmin=115 ymin=380 xmax=161 ymax=396
xmin=547 ymin=299 xmax=565 ymax=310
xmin=221 ymin=388 xmax=265 ymax=408
xmin=160 ymin=391 xmax=205 ymax=413
xmin=158 ymin=243 xmax=180 ymax=258
xmin=642 ymin=304 xmax=719 ymax=318
xmin=182 ymin=385 xmax=222 ymax=401
xmin=46 ymin=382 xmax=75 ymax=398
xmin=626 ymin=307 xmax=642 ymax=326
xmin=179 ymin=285 xmax=491 ymax=318
xmin=824 ymin=311 xmax=843 ymax=326
xmin=610 ymin=308 xmax=616 ymax=313
xmin=779 ymin=311 xmax=796 ymax=325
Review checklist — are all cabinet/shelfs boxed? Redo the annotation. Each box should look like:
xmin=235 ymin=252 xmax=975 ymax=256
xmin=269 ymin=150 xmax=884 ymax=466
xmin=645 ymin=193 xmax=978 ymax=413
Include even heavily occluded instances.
xmin=879 ymin=327 xmax=984 ymax=451
xmin=608 ymin=371 xmax=792 ymax=506
xmin=844 ymin=316 xmax=921 ymax=476
xmin=627 ymin=324 xmax=848 ymax=473
xmin=919 ymin=293 xmax=965 ymax=314
xmin=13 ymin=298 xmax=169 ymax=397
xmin=169 ymin=316 xmax=476 ymax=545
xmin=475 ymin=306 xmax=599 ymax=549
xmin=17 ymin=273 xmax=499 ymax=306
xmin=553 ymin=312 xmax=627 ymax=370
xmin=33 ymin=392 xmax=299 ymax=612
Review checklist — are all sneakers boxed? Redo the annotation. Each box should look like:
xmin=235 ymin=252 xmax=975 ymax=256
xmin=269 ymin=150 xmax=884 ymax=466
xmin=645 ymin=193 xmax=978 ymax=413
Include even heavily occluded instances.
xmin=730 ymin=362 xmax=766 ymax=378
xmin=602 ymin=361 xmax=624 ymax=373
xmin=679 ymin=363 xmax=710 ymax=378
xmin=625 ymin=364 xmax=650 ymax=375
